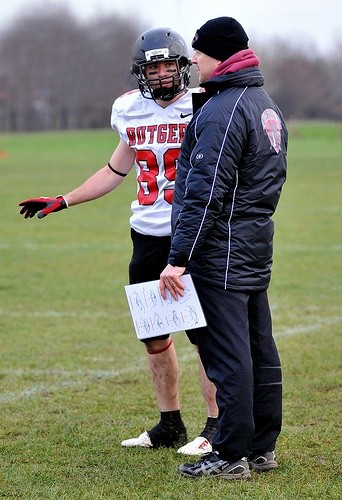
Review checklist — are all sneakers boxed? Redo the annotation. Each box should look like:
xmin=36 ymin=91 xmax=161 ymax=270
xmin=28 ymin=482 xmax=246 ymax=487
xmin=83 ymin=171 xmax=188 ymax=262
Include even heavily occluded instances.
xmin=179 ymin=451 xmax=251 ymax=480
xmin=247 ymin=451 xmax=278 ymax=471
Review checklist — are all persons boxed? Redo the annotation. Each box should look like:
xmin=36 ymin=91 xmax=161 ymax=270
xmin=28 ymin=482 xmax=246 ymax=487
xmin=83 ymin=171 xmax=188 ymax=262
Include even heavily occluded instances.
xmin=158 ymin=16 xmax=289 ymax=479
xmin=19 ymin=26 xmax=221 ymax=457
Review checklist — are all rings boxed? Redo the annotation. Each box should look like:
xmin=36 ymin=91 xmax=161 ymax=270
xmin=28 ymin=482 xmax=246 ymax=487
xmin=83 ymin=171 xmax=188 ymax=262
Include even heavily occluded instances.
xmin=171 ymin=282 xmax=175 ymax=286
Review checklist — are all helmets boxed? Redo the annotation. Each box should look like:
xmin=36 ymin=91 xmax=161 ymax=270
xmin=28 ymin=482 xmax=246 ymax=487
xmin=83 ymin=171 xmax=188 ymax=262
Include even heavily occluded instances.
xmin=129 ymin=27 xmax=193 ymax=101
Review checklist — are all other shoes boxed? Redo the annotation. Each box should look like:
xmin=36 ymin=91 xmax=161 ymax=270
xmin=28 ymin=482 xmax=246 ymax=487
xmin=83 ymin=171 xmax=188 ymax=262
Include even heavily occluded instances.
xmin=177 ymin=423 xmax=219 ymax=455
xmin=121 ymin=424 xmax=187 ymax=447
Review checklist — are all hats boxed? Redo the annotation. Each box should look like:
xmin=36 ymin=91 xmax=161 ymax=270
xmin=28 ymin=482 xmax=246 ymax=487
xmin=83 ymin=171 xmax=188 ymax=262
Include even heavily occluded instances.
xmin=192 ymin=17 xmax=249 ymax=62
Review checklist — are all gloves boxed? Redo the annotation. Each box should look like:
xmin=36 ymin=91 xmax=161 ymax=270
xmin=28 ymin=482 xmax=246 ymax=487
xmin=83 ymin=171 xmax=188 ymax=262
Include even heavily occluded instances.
xmin=18 ymin=195 xmax=68 ymax=219
